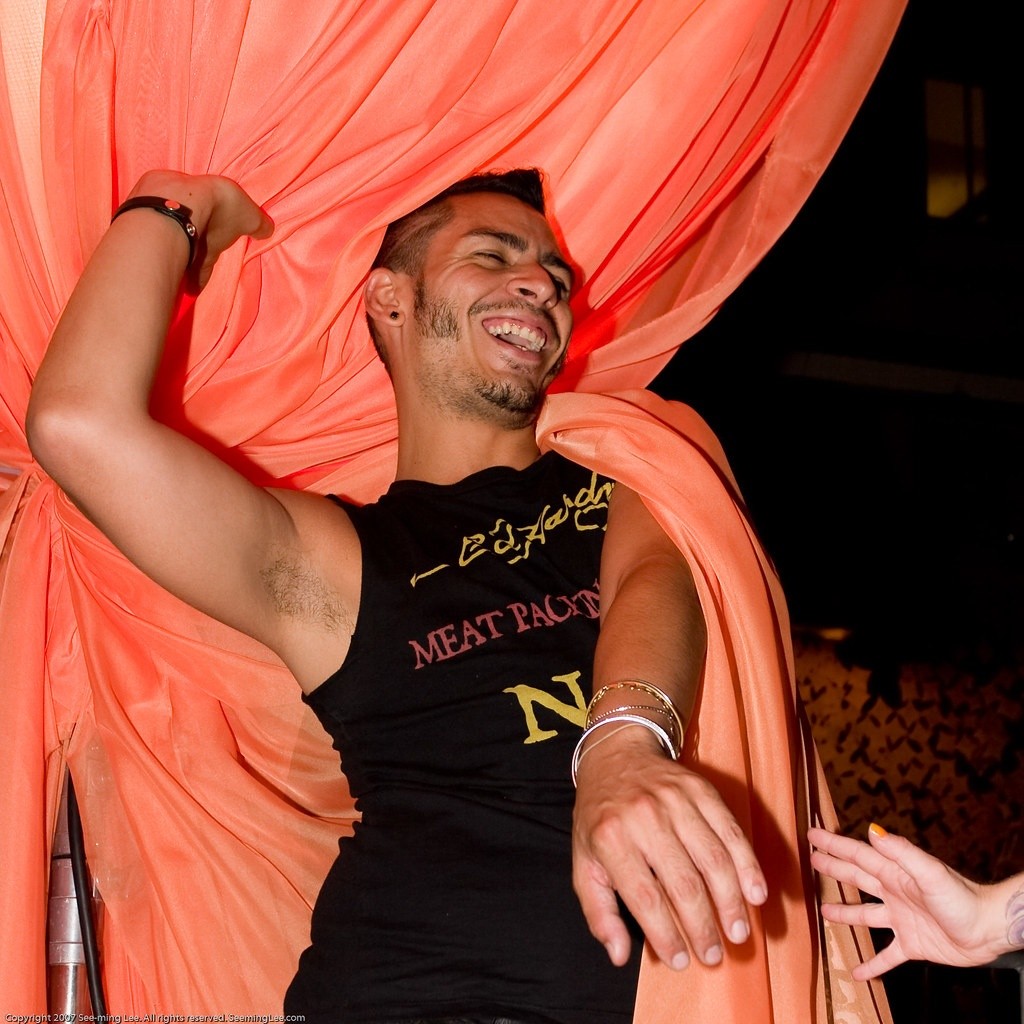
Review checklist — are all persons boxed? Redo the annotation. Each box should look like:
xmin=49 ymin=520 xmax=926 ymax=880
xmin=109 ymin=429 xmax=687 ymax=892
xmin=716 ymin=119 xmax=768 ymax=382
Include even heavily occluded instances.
xmin=26 ymin=169 xmax=770 ymax=1024
xmin=808 ymin=822 xmax=1024 ymax=982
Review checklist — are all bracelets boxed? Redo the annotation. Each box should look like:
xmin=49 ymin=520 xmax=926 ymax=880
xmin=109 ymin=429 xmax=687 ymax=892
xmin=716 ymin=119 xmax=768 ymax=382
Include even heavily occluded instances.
xmin=571 ymin=679 xmax=684 ymax=787
xmin=111 ymin=196 xmax=198 ymax=270
